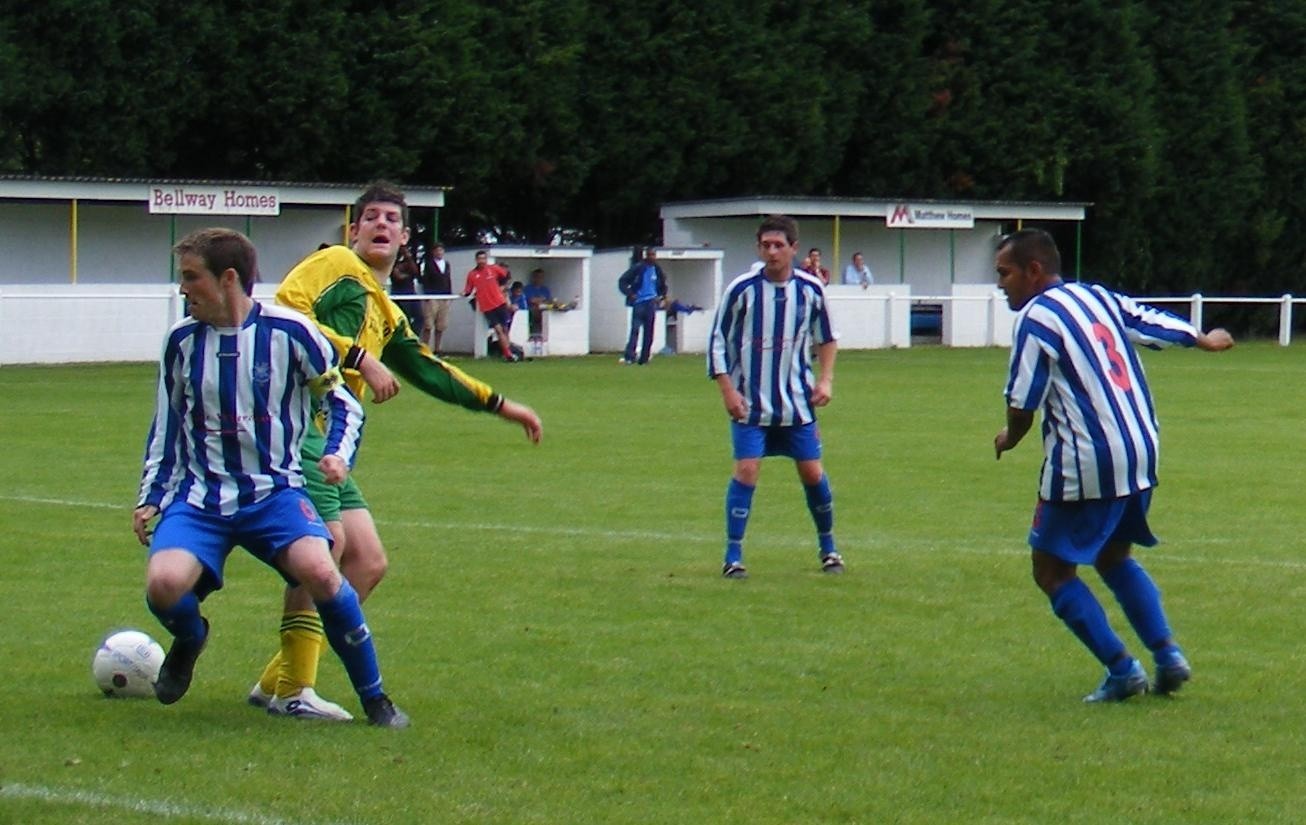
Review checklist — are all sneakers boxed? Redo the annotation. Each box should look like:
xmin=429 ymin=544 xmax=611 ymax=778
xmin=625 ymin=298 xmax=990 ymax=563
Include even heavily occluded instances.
xmin=266 ymin=686 xmax=353 ymax=722
xmin=246 ymin=681 xmax=272 ymax=708
xmin=1080 ymin=659 xmax=1150 ymax=703
xmin=364 ymin=695 xmax=409 ymax=727
xmin=822 ymin=551 xmax=845 ymax=573
xmin=154 ymin=617 xmax=211 ymax=705
xmin=721 ymin=561 xmax=746 ymax=577
xmin=1150 ymin=644 xmax=1190 ymax=695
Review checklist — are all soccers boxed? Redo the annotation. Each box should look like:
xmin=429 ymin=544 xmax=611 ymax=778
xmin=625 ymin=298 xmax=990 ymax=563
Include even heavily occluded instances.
xmin=92 ymin=628 xmax=167 ymax=700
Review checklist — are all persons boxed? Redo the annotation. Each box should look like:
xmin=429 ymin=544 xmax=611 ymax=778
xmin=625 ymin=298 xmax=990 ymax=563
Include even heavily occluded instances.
xmin=317 ymin=243 xmax=553 ymax=362
xmin=994 ymin=229 xmax=1235 ymax=703
xmin=842 ymin=252 xmax=874 ymax=289
xmin=249 ymin=180 xmax=541 ymax=721
xmin=800 ymin=248 xmax=830 ymax=285
xmin=618 ymin=247 xmax=668 ymax=365
xmin=706 ymin=215 xmax=846 ymax=578
xmin=132 ymin=227 xmax=409 ymax=727
